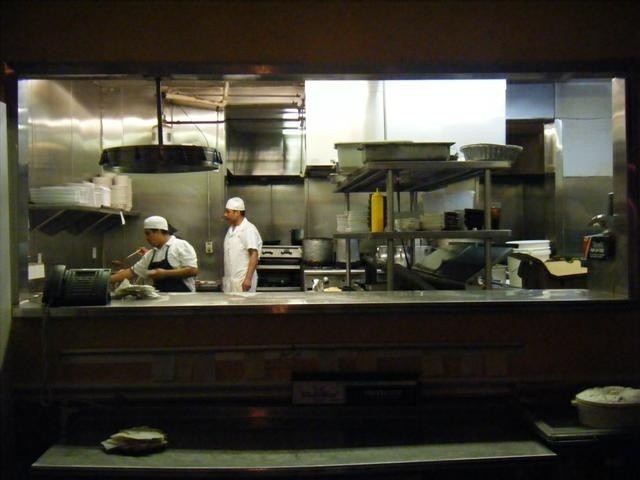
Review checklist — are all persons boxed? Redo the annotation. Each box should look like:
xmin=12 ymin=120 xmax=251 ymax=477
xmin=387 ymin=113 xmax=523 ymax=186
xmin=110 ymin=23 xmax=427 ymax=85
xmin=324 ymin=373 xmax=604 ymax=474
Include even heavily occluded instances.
xmin=222 ymin=196 xmax=263 ymax=293
xmin=136 ymin=223 xmax=182 ymax=256
xmin=111 ymin=215 xmax=199 ymax=293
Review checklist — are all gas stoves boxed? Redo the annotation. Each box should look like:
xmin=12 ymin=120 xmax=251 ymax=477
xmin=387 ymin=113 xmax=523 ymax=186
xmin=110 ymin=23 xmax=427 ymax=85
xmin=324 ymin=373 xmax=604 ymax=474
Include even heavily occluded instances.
xmin=302 ymin=262 xmax=364 ymax=270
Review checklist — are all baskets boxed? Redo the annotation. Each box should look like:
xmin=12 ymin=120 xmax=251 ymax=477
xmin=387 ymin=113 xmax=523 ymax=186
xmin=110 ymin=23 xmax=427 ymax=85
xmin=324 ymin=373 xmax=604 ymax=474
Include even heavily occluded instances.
xmin=460 ymin=143 xmax=523 ymax=163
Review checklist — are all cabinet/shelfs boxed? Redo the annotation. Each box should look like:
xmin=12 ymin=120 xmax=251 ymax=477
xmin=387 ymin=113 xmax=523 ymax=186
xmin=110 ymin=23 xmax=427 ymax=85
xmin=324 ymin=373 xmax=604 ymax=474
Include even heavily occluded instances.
xmin=332 ymin=160 xmax=513 ymax=289
xmin=304 ymin=266 xmax=367 ymax=291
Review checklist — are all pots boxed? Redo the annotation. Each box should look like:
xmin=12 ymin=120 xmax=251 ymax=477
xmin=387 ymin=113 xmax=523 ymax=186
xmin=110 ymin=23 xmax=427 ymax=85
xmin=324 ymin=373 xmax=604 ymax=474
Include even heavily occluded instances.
xmin=571 ymin=385 xmax=640 ymax=428
xmin=291 ymin=228 xmax=305 ymax=245
xmin=336 ymin=238 xmax=361 ymax=263
xmin=302 ymin=237 xmax=334 ymax=264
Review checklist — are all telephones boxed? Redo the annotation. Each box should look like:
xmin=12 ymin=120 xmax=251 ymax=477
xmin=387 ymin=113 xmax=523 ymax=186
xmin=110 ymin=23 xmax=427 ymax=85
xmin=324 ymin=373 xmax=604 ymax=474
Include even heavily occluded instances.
xmin=41 ymin=265 xmax=111 ymax=307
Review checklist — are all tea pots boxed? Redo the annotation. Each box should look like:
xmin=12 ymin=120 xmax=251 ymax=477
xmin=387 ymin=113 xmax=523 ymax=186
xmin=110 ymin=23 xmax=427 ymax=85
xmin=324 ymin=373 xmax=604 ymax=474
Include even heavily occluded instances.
xmin=393 ymin=245 xmax=410 ymax=268
xmin=375 ymin=245 xmax=388 ymax=265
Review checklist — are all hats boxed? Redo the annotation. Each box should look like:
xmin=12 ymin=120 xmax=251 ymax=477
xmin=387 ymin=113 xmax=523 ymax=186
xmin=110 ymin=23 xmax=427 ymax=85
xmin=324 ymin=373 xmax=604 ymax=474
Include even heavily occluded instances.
xmin=225 ymin=197 xmax=246 ymax=211
xmin=143 ymin=216 xmax=168 ymax=230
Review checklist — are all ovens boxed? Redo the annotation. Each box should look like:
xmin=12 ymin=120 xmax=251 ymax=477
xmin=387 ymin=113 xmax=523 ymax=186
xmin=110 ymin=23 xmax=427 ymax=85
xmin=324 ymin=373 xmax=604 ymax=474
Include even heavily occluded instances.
xmin=255 ymin=245 xmax=302 ymax=291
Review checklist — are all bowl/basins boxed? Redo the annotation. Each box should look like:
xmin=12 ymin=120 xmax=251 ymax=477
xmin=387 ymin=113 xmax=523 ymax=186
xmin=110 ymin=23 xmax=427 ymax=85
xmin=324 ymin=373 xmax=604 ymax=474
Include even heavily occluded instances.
xmin=444 ymin=211 xmax=464 ymax=229
xmin=464 ymin=208 xmax=485 ymax=230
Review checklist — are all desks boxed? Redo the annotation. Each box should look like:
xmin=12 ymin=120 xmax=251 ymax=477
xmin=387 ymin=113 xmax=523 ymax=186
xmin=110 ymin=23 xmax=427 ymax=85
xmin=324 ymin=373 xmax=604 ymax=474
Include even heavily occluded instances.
xmin=30 ymin=440 xmax=559 ymax=478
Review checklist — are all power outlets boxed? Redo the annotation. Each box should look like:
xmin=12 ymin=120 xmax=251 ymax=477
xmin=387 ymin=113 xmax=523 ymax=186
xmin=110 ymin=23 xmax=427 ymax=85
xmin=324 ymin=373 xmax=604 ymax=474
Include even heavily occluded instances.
xmin=205 ymin=240 xmax=213 ymax=254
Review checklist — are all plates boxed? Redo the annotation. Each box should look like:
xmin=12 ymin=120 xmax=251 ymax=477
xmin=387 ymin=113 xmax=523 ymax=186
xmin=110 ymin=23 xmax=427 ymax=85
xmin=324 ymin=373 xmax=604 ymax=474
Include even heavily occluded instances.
xmin=420 ymin=213 xmax=444 ymax=231
xmin=93 ymin=176 xmax=112 ymax=207
xmin=393 ymin=218 xmax=419 ymax=231
xmin=29 ymin=182 xmax=95 ymax=207
xmin=114 ymin=176 xmax=133 ymax=212
xmin=336 ymin=210 xmax=370 ymax=232
xmin=111 ymin=185 xmax=128 ymax=210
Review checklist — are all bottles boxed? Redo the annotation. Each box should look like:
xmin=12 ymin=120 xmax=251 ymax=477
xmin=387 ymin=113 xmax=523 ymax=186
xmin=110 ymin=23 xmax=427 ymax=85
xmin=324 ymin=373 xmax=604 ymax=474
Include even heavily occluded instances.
xmin=371 ymin=188 xmax=383 ymax=232
xmin=491 ymin=202 xmax=501 ymax=230
